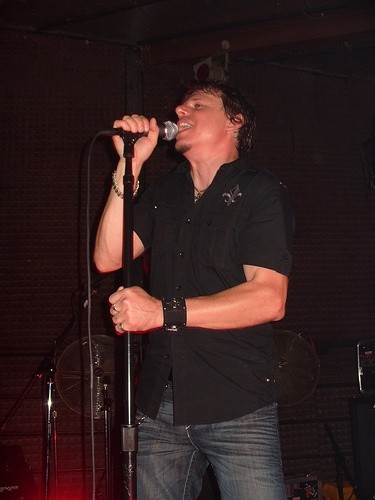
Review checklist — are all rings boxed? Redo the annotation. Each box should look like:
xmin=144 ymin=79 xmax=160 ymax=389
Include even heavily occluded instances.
xmin=119 ymin=323 xmax=124 ymax=332
xmin=112 ymin=304 xmax=118 ymax=313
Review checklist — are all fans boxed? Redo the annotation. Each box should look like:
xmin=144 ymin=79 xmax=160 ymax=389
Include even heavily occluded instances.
xmin=54 ymin=334 xmax=139 ymax=500
xmin=272 ymin=329 xmax=322 ymax=408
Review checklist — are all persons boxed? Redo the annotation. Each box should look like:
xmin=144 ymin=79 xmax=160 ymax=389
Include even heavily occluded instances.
xmin=93 ymin=79 xmax=294 ymax=500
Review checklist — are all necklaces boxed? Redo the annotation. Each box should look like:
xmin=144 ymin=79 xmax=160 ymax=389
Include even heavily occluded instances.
xmin=192 ymin=186 xmax=206 ymax=201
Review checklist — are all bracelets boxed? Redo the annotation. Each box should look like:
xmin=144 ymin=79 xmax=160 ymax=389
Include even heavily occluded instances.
xmin=113 ymin=169 xmax=140 ymax=198
xmin=162 ymin=296 xmax=186 ymax=331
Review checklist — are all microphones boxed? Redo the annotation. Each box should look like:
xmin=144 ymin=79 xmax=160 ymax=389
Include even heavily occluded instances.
xmin=345 ymin=472 xmax=359 ymax=497
xmin=76 ymin=286 xmax=98 ymax=316
xmin=102 ymin=375 xmax=110 ymax=411
xmin=101 ymin=121 xmax=179 ymax=141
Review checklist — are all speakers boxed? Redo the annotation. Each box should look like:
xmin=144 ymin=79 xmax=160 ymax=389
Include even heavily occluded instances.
xmin=350 ymin=396 xmax=375 ymax=500
